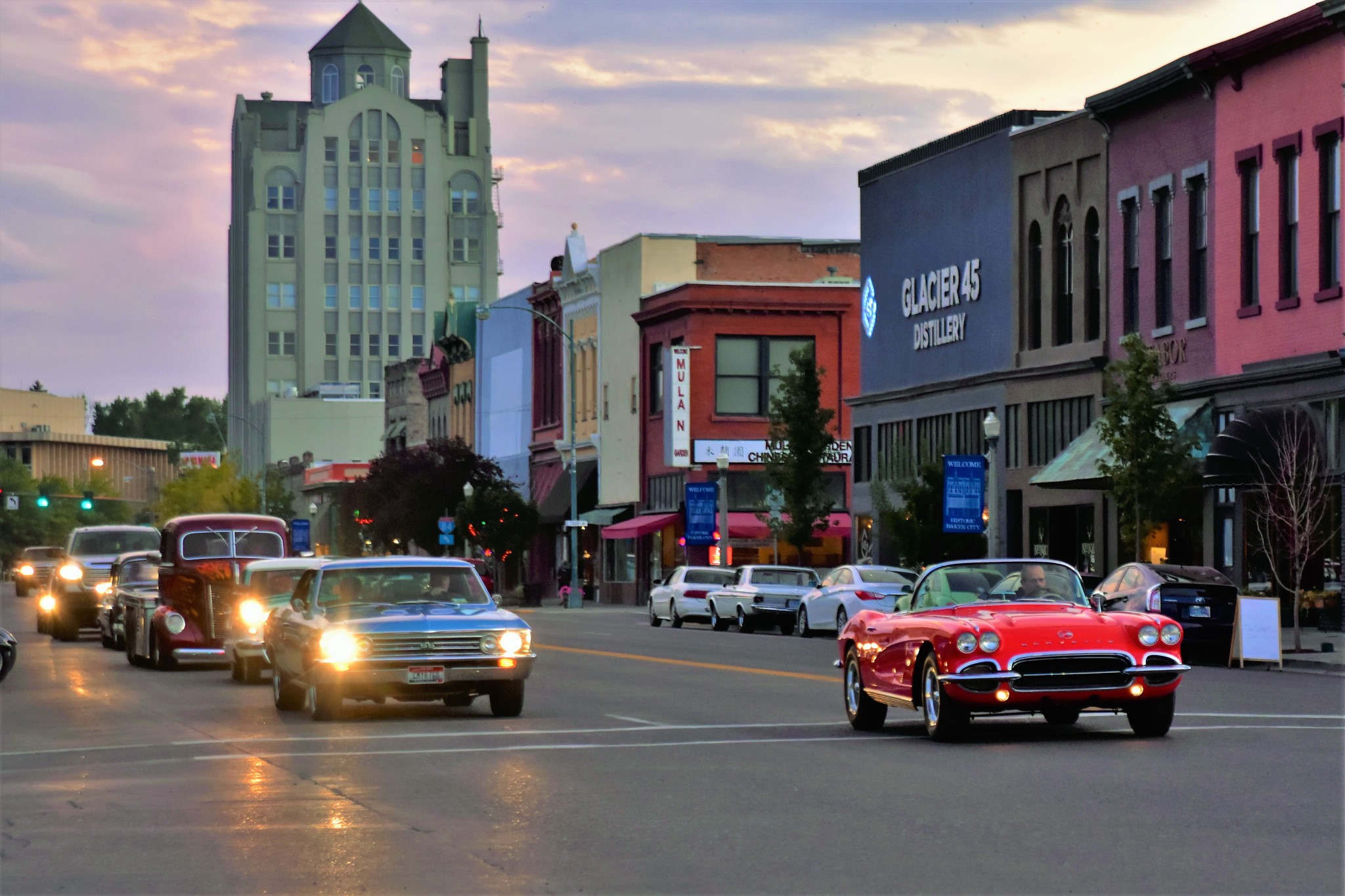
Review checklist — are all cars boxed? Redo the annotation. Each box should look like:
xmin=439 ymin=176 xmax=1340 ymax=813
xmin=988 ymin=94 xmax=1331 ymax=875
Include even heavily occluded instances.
xmin=646 ymin=565 xmax=743 ymax=628
xmin=261 ymin=555 xmax=537 ymax=719
xmin=12 ymin=544 xmax=66 ymax=597
xmin=93 ymin=549 xmax=165 ymax=668
xmin=892 ymin=567 xmax=1007 ymax=613
xmin=975 ymin=570 xmax=1080 ymax=606
xmin=794 ymin=563 xmax=930 ymax=638
xmin=143 ymin=512 xmax=291 ymax=670
xmin=705 ymin=563 xmax=823 ymax=636
xmin=223 ymin=556 xmax=338 ymax=687
xmin=1087 ymin=562 xmax=1245 ymax=664
xmin=832 ymin=557 xmax=1190 ymax=745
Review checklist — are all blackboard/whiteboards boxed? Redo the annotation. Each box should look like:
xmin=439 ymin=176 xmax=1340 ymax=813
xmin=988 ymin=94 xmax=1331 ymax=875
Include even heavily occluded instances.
xmin=1229 ymin=594 xmax=1282 ymax=662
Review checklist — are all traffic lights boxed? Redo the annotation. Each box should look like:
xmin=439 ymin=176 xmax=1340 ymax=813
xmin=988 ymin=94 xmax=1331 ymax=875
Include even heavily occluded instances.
xmin=37 ymin=483 xmax=49 ymax=508
xmin=81 ymin=491 xmax=93 ymax=509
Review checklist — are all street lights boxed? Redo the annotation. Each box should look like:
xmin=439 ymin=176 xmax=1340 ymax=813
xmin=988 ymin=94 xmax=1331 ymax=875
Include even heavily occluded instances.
xmin=717 ymin=452 xmax=731 ymax=569
xmin=462 ymin=481 xmax=475 ymax=558
xmin=309 ymin=502 xmax=318 ymax=557
xmin=981 ymin=410 xmax=1002 ymax=567
xmin=474 ymin=299 xmax=584 ymax=608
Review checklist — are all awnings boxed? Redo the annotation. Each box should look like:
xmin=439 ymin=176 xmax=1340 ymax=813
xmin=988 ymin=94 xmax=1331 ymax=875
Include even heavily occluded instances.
xmin=380 ymin=420 xmax=406 ymax=441
xmin=715 ymin=513 xmax=852 ymax=540
xmin=580 ymin=508 xmax=632 ymax=527
xmin=1031 ymin=396 xmax=1215 ymax=489
xmin=1202 ymin=402 xmax=1317 ymax=488
xmin=601 ymin=514 xmax=678 ymax=539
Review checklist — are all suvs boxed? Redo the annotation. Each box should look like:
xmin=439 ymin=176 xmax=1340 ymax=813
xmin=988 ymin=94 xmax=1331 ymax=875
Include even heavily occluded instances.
xmin=35 ymin=524 xmax=162 ymax=642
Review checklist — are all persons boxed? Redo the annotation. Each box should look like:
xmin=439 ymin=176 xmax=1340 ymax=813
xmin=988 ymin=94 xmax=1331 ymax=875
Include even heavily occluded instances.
xmin=557 ymin=560 xmax=572 ymax=606
xmin=977 ymin=563 xmax=1073 ymax=603
xmin=424 ymin=568 xmax=469 ymax=605
xmin=336 ymin=576 xmax=361 ymax=602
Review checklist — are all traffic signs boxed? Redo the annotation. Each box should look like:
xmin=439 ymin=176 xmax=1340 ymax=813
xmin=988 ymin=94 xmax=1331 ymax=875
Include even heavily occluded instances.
xmin=6 ymin=496 xmax=19 ymax=510
